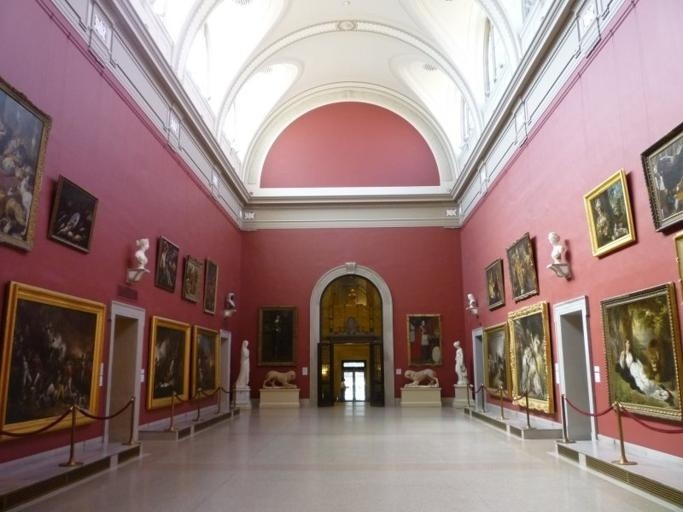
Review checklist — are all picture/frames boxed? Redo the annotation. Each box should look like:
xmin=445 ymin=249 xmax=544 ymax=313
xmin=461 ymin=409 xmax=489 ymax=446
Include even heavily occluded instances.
xmin=1 ymin=77 xmax=54 ymax=257
xmin=146 ymin=314 xmax=192 ymax=409
xmin=256 ymin=305 xmax=298 ymax=368
xmin=202 ymin=256 xmax=219 ymax=314
xmin=192 ymin=323 xmax=220 ymax=401
xmin=47 ymin=172 xmax=101 ymax=255
xmin=504 ymin=231 xmax=540 ymax=303
xmin=484 ymin=256 xmax=507 ymax=310
xmin=598 ymin=280 xmax=682 ymax=422
xmin=406 ymin=313 xmax=444 ymax=368
xmin=154 ymin=234 xmax=182 ymax=293
xmin=506 ymin=299 xmax=556 ymax=416
xmin=483 ymin=320 xmax=511 ymax=404
xmin=639 ymin=120 xmax=682 ymax=233
xmin=580 ymin=166 xmax=638 ymax=257
xmin=182 ymin=252 xmax=202 ymax=305
xmin=2 ymin=279 xmax=109 ymax=438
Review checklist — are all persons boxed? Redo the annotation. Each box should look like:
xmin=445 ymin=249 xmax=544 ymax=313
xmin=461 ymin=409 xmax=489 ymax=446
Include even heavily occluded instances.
xmin=521 ymin=334 xmax=545 ymax=395
xmin=236 ymin=341 xmax=250 ymax=387
xmin=468 ymin=294 xmax=477 ymax=308
xmin=548 ymin=232 xmax=565 ymax=264
xmin=596 ymin=203 xmax=611 ymax=237
xmin=340 ymin=377 xmax=347 ymax=402
xmin=135 ymin=238 xmax=150 ymax=268
xmin=453 ymin=342 xmax=469 ymax=385
xmin=262 ymin=315 xmax=282 ymax=361
xmin=17 ymin=346 xmax=78 ymax=406
xmin=418 ymin=320 xmax=429 ymax=361
xmin=619 ymin=338 xmax=635 ymax=369
